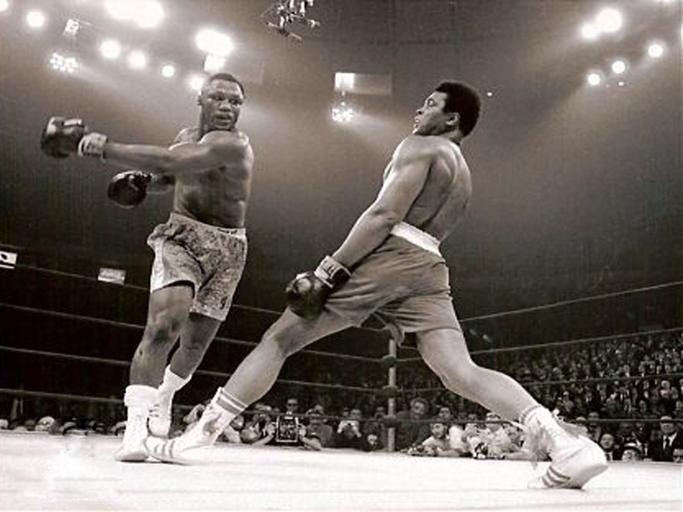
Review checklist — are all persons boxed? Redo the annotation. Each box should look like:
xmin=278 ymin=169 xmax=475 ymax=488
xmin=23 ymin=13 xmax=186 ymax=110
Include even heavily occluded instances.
xmin=40 ymin=72 xmax=257 ymax=463
xmin=0 ymin=330 xmax=683 ymax=463
xmin=141 ymin=76 xmax=608 ymax=490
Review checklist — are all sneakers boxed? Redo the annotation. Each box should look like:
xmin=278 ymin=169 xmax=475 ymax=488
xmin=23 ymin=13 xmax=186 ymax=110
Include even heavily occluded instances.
xmin=114 ymin=386 xmax=218 ymax=467
xmin=526 ymin=436 xmax=609 ymax=491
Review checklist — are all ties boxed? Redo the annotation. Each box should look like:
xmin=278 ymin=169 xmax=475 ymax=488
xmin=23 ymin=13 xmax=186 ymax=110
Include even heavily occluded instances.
xmin=665 ymin=438 xmax=670 ymax=449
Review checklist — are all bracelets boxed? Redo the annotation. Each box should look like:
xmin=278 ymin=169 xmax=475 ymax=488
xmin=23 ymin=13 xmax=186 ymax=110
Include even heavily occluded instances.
xmin=146 ymin=171 xmax=171 ymax=196
xmin=313 ymin=250 xmax=354 ymax=290
xmin=77 ymin=131 xmax=108 ymax=163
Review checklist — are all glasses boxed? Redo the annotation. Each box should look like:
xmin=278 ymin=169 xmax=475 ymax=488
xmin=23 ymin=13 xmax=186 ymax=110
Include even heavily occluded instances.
xmin=287 ymin=403 xmax=299 ymax=407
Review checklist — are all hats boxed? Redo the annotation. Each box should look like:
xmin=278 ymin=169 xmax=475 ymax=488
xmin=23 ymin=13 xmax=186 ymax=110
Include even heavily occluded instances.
xmin=622 ymin=442 xmax=642 ymax=455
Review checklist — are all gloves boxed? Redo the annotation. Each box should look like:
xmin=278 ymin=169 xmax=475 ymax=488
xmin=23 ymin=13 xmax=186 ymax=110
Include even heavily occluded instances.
xmin=107 ymin=169 xmax=155 ymax=210
xmin=281 ymin=254 xmax=353 ymax=322
xmin=40 ymin=115 xmax=108 ymax=164
